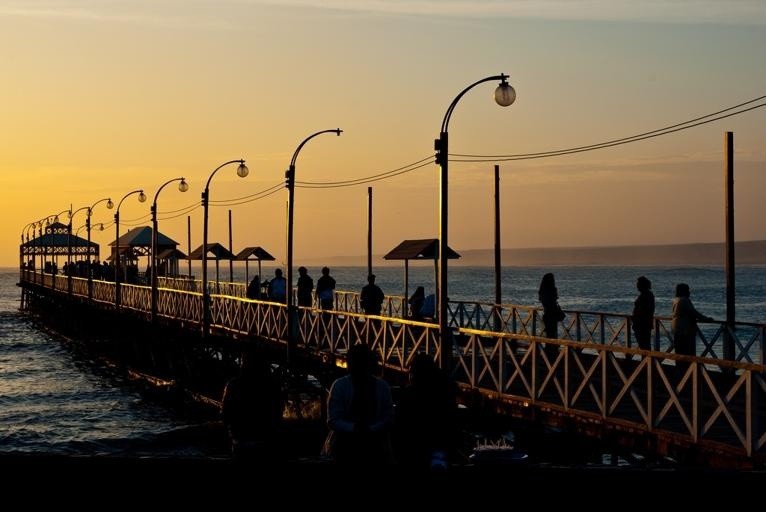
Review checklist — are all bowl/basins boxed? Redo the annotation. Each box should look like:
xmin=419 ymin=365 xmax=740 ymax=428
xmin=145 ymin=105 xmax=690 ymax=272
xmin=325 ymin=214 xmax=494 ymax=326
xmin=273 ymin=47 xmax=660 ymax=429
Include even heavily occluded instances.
xmin=474 ymin=446 xmax=514 ymax=457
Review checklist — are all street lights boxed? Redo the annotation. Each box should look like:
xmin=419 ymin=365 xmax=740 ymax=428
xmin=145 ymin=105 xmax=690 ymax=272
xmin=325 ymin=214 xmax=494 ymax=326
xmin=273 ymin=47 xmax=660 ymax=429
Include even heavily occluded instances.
xmin=148 ymin=175 xmax=190 ymax=318
xmin=17 ymin=196 xmax=113 ymax=300
xmin=201 ymin=158 xmax=250 ymax=339
xmin=435 ymin=68 xmax=522 ymax=377
xmin=113 ymin=188 xmax=147 ymax=311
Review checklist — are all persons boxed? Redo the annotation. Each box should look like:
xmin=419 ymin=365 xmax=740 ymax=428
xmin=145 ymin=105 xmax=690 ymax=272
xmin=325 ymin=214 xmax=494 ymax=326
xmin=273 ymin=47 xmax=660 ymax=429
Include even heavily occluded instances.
xmin=314 ymin=266 xmax=336 ymax=310
xmin=359 ymin=275 xmax=384 ymax=315
xmin=671 ymin=284 xmax=713 ymax=357
xmin=218 ymin=361 xmax=285 ymax=460
xmin=408 ymin=286 xmax=434 ymax=317
xmin=23 ymin=259 xmax=138 ymax=285
xmin=246 ymin=268 xmax=286 ymax=304
xmin=297 ymin=266 xmax=313 ymax=307
xmin=631 ymin=276 xmax=655 ymax=350
xmin=326 ymin=344 xmax=467 ymax=470
xmin=538 ymin=273 xmax=566 ymax=338
